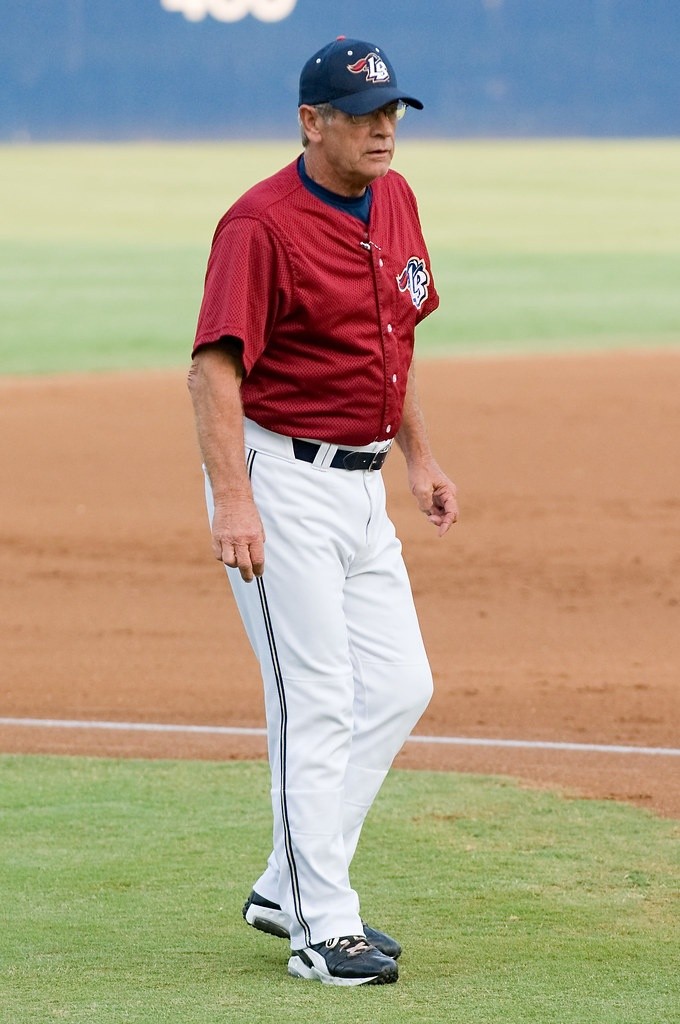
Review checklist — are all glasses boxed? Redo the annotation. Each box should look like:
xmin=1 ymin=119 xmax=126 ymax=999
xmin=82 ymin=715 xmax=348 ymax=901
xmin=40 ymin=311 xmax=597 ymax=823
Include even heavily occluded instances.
xmin=313 ymin=100 xmax=409 ymax=125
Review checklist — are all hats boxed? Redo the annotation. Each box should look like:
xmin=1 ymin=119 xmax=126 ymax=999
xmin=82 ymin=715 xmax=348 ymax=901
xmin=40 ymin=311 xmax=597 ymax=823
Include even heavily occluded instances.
xmin=298 ymin=36 xmax=423 ymax=115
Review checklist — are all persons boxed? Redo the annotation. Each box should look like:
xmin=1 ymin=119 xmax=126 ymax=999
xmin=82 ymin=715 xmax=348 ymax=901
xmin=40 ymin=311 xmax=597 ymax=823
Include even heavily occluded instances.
xmin=187 ymin=36 xmax=461 ymax=988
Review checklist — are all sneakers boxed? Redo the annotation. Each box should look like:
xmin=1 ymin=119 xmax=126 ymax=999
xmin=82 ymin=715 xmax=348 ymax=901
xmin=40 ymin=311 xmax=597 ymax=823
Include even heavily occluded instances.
xmin=287 ymin=935 xmax=399 ymax=986
xmin=242 ymin=890 xmax=402 ymax=961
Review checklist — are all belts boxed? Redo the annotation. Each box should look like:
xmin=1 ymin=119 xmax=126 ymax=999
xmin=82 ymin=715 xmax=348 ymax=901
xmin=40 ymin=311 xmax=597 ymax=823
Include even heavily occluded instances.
xmin=293 ymin=438 xmax=391 ymax=472
